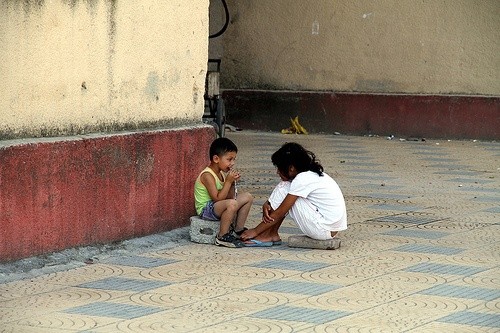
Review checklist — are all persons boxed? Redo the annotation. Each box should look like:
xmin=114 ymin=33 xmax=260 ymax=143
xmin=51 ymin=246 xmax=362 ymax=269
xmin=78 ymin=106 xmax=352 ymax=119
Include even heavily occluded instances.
xmin=238 ymin=142 xmax=348 ymax=248
xmin=194 ymin=137 xmax=253 ymax=248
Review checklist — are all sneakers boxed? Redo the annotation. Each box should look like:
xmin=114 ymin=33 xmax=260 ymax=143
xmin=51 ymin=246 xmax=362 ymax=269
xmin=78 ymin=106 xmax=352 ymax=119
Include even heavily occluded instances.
xmin=216 ymin=233 xmax=243 ymax=248
xmin=233 ymin=227 xmax=248 ymax=238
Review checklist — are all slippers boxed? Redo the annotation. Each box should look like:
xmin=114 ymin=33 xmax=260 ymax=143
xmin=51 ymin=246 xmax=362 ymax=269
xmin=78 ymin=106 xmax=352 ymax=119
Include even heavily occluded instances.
xmin=244 ymin=239 xmax=282 ymax=246
